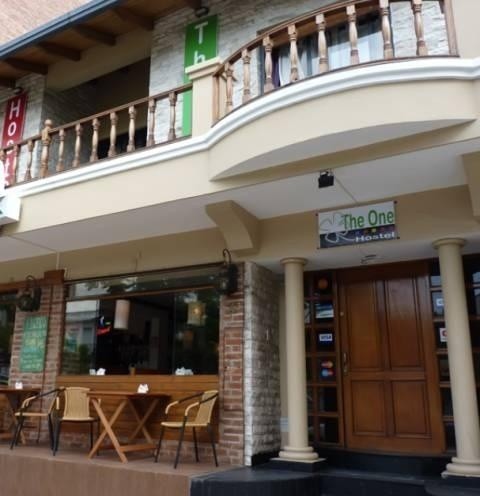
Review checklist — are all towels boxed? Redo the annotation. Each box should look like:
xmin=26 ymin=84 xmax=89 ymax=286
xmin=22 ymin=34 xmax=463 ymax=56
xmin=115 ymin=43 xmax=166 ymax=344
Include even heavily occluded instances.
xmin=175 ymin=366 xmax=194 ymax=377
xmin=15 ymin=378 xmax=23 ymax=390
xmin=137 ymin=384 xmax=149 ymax=393
xmin=96 ymin=367 xmax=106 ymax=376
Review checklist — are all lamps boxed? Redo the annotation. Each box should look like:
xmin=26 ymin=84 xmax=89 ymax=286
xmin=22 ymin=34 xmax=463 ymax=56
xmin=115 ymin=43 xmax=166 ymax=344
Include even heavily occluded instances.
xmin=113 ymin=252 xmax=141 ymax=331
xmin=215 ymin=249 xmax=238 ymax=294
xmin=12 ymin=87 xmax=24 ymax=94
xmin=195 ymin=7 xmax=208 ymax=17
xmin=16 ymin=275 xmax=41 ymax=312
xmin=186 ymin=301 xmax=206 ymax=328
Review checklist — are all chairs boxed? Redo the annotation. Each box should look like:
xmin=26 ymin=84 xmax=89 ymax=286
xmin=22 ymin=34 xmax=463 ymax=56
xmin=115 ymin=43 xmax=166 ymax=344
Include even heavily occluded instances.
xmin=52 ymin=386 xmax=102 ymax=458
xmin=155 ymin=390 xmax=220 ymax=469
xmin=10 ymin=386 xmax=65 ymax=450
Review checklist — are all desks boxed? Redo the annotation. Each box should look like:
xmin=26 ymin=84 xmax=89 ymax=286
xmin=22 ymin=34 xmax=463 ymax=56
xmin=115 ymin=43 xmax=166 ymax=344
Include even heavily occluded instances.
xmin=0 ymin=385 xmax=41 ymax=450
xmin=82 ymin=391 xmax=171 ymax=465
